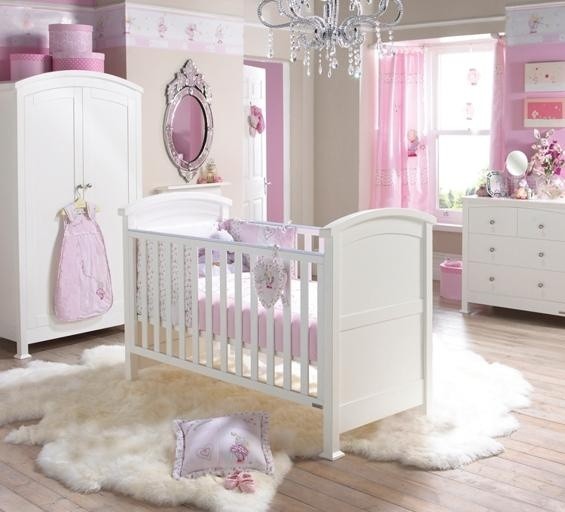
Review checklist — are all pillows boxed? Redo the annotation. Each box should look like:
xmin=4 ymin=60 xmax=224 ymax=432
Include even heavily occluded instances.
xmin=168 ymin=411 xmax=276 ymax=480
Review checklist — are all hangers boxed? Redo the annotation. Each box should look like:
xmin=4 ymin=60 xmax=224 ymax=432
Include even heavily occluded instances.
xmin=59 ymin=185 xmax=101 ymax=219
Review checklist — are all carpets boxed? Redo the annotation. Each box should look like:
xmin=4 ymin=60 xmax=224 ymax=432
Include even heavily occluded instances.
xmin=1 ymin=328 xmax=535 ymax=512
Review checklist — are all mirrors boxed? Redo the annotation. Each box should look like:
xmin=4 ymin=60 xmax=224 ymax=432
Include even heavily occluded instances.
xmin=162 ymin=58 xmax=215 ymax=183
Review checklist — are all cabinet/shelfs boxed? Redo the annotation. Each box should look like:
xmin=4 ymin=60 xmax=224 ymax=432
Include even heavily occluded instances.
xmin=1 ymin=70 xmax=145 ymax=361
xmin=459 ymin=195 xmax=565 ymax=319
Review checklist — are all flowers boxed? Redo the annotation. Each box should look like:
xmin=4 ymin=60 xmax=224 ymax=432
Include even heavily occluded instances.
xmin=526 ymin=128 xmax=565 ymax=183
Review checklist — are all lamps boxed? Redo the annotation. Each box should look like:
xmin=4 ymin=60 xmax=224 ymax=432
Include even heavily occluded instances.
xmin=258 ymin=0 xmax=404 ymax=79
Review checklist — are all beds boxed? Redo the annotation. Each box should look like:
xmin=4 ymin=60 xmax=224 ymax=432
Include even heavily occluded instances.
xmin=119 ymin=190 xmax=437 ymax=462
